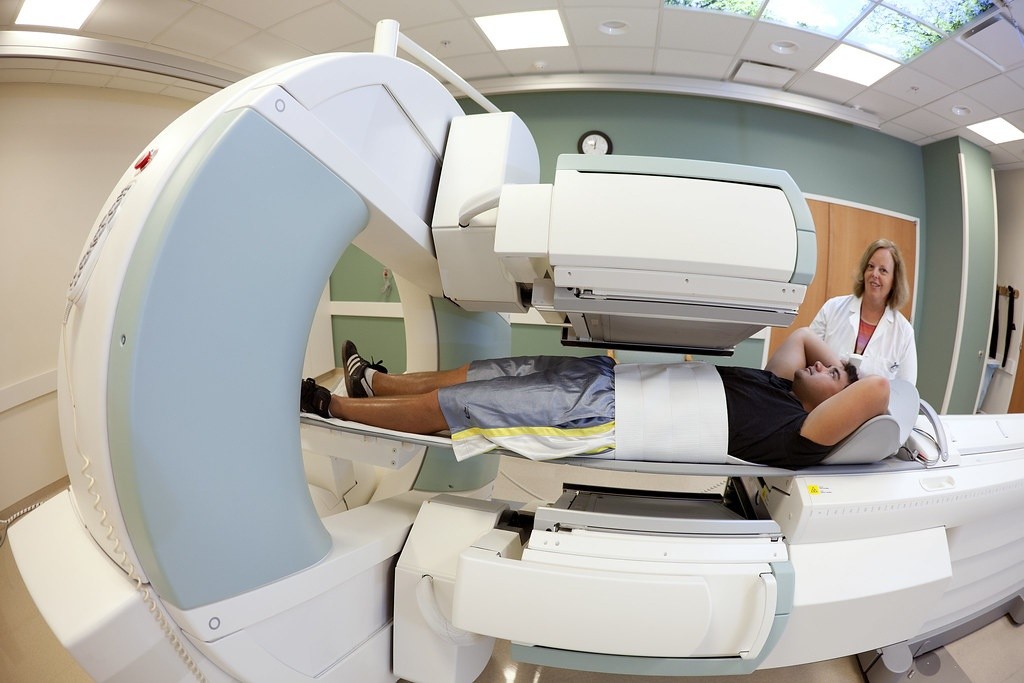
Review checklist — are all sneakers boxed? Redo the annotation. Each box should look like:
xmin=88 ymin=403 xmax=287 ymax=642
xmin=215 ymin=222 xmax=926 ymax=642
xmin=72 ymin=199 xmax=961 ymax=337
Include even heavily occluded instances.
xmin=301 ymin=377 xmax=336 ymax=424
xmin=340 ymin=336 xmax=388 ymax=399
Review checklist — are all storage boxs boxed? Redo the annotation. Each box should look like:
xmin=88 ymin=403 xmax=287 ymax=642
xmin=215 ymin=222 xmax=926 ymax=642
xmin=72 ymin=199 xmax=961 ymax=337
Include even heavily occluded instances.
xmin=976 ymin=357 xmax=1001 ymax=412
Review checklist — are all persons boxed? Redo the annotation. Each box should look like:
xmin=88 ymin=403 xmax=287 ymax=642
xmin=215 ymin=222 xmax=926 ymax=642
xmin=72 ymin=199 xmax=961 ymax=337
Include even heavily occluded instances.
xmin=300 ymin=328 xmax=891 ymax=471
xmin=809 ymin=239 xmax=918 ymax=387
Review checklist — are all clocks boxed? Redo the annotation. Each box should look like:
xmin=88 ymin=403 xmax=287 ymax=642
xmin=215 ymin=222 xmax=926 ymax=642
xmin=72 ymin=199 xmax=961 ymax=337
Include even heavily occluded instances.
xmin=577 ymin=131 xmax=613 ymax=155
